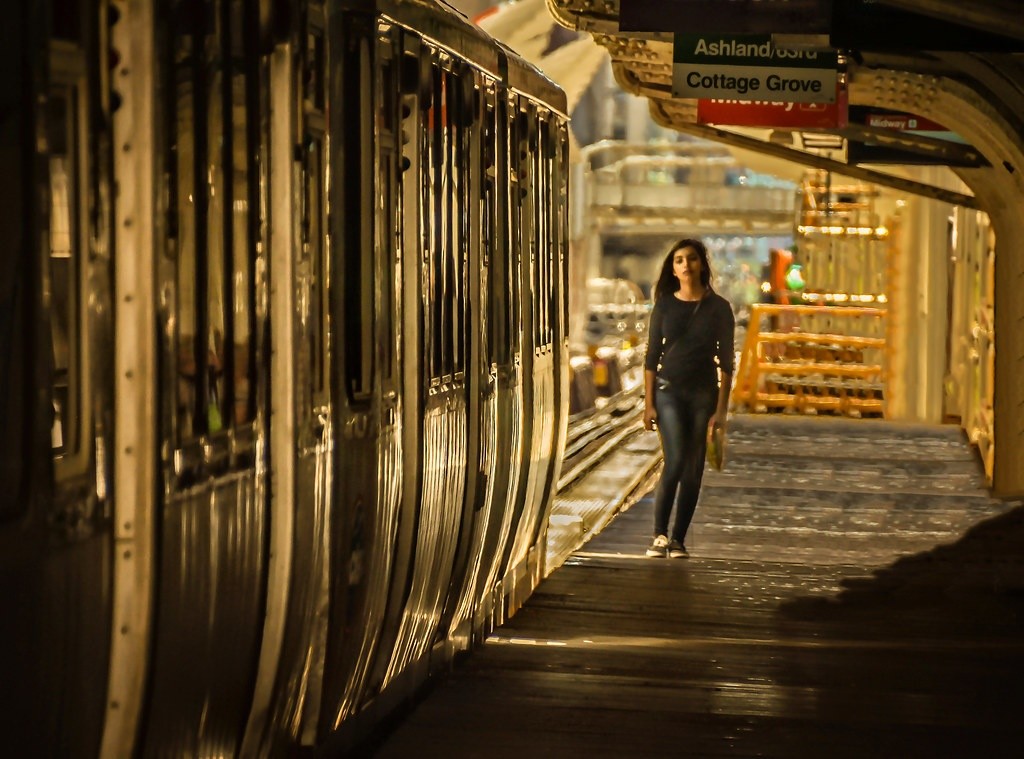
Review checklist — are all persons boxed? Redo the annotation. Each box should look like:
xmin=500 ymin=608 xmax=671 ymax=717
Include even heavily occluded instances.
xmin=643 ymin=239 xmax=735 ymax=559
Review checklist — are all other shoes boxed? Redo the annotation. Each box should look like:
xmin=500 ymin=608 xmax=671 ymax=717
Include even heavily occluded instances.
xmin=669 ymin=539 xmax=689 ymax=558
xmin=646 ymin=535 xmax=668 ymax=557
